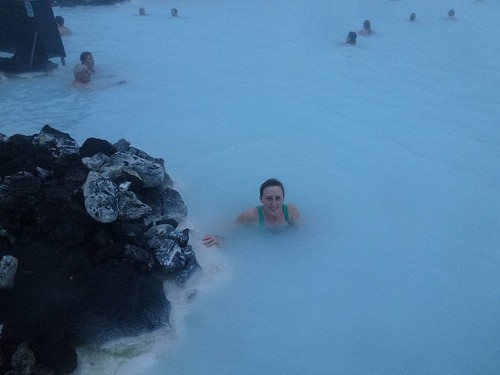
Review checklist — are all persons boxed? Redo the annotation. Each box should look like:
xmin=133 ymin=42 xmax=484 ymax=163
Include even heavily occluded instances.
xmin=447 ymin=9 xmax=455 ymax=17
xmin=409 ymin=12 xmax=416 ymax=21
xmin=364 ymin=19 xmax=375 ymax=34
xmin=54 ymin=15 xmax=72 ymax=36
xmin=80 ymin=52 xmax=96 ymax=73
xmin=71 ymin=63 xmax=129 ymax=93
xmin=171 ymin=8 xmax=177 ymax=17
xmin=344 ymin=32 xmax=357 ymax=46
xmin=138 ymin=7 xmax=146 ymax=16
xmin=201 ymin=177 xmax=300 ymax=249
